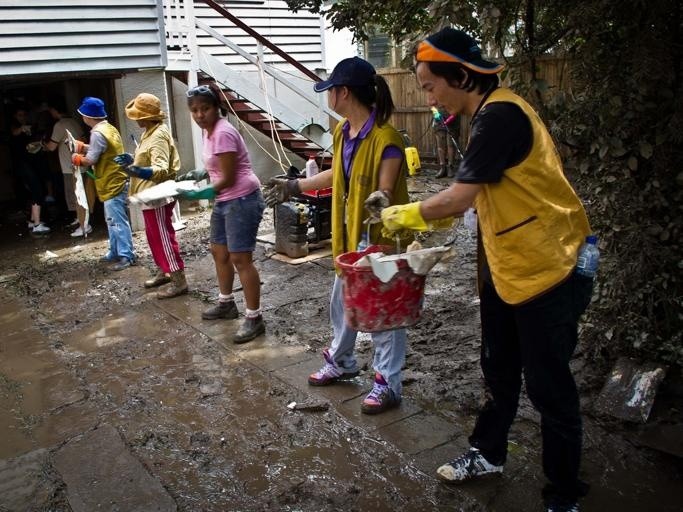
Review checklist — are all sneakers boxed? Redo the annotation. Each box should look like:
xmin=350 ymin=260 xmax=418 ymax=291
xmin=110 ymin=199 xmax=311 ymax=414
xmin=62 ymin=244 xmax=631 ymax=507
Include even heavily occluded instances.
xmin=308 ymin=346 xmax=361 ymax=386
xmin=202 ymin=300 xmax=240 ymax=321
xmin=28 ymin=218 xmax=92 ymax=237
xmin=360 ymin=372 xmax=403 ymax=415
xmin=434 ymin=443 xmax=505 ymax=488
xmin=233 ymin=311 xmax=267 ymax=344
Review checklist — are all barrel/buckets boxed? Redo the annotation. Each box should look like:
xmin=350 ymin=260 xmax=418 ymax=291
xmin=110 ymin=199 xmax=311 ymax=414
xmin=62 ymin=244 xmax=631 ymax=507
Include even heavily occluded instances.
xmin=335 ymin=216 xmax=426 ymax=334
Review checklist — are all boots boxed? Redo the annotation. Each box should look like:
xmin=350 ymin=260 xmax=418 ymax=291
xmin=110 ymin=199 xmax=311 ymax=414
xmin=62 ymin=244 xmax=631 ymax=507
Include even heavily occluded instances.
xmin=158 ymin=269 xmax=188 ymax=299
xmin=144 ymin=270 xmax=170 ymax=288
xmin=447 ymin=164 xmax=455 ymax=178
xmin=435 ymin=164 xmax=447 ymax=179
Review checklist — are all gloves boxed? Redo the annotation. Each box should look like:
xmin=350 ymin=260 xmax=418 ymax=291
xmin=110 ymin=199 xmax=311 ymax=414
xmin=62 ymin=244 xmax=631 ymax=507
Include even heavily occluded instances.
xmin=113 ymin=152 xmax=134 ymax=167
xmin=431 ymin=106 xmax=441 ymax=121
xmin=362 ymin=189 xmax=454 ymax=240
xmin=71 ymin=153 xmax=82 ymax=166
xmin=124 ymin=166 xmax=154 ymax=181
xmin=261 ymin=175 xmax=301 ymax=209
xmin=64 ymin=139 xmax=85 ymax=153
xmin=176 ymin=185 xmax=216 ymax=201
xmin=175 ymin=169 xmax=209 ymax=182
xmin=439 ymin=114 xmax=455 ymax=128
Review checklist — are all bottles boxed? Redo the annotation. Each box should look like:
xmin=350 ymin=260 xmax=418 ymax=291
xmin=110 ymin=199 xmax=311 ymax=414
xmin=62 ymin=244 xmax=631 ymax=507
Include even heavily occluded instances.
xmin=305 ymin=154 xmax=318 ymax=178
xmin=576 ymin=235 xmax=601 ymax=277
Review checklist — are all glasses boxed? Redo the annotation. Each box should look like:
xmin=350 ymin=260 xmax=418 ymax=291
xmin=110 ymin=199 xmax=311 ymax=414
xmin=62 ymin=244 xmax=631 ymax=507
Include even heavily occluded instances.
xmin=185 ymin=83 xmax=217 ymax=99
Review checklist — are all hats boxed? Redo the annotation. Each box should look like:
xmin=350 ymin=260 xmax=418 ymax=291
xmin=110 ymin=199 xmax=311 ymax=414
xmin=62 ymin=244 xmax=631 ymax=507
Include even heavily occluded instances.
xmin=124 ymin=91 xmax=168 ymax=123
xmin=76 ymin=96 xmax=107 ymax=120
xmin=313 ymin=55 xmax=377 ymax=94
xmin=415 ymin=27 xmax=506 ymax=77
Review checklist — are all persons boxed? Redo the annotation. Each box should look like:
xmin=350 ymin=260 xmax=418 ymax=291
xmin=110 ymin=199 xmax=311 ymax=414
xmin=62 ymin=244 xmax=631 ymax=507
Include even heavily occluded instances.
xmin=8 ymin=98 xmax=93 ymax=237
xmin=67 ymin=97 xmax=135 ymax=271
xmin=431 ymin=105 xmax=461 ymax=178
xmin=114 ymin=93 xmax=188 ymax=299
xmin=174 ymin=85 xmax=266 ymax=344
xmin=264 ymin=56 xmax=411 ymax=414
xmin=380 ymin=26 xmax=599 ymax=512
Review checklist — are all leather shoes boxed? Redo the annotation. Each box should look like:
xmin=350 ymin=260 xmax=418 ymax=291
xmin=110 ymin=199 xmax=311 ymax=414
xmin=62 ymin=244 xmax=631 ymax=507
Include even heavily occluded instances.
xmin=111 ymin=256 xmax=131 ymax=271
